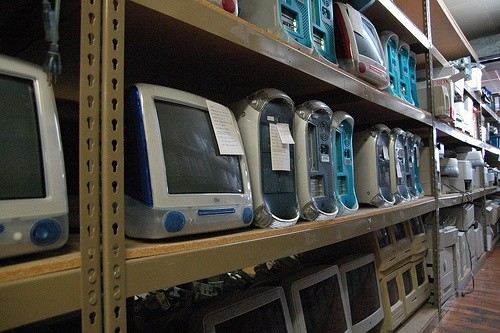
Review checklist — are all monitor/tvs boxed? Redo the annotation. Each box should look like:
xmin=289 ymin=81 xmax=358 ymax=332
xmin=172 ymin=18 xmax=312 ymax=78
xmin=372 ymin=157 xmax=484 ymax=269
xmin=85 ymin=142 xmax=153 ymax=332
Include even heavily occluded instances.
xmin=332 ymin=2 xmax=390 ymax=90
xmin=118 ymin=82 xmax=253 ymax=241
xmin=0 ymin=55 xmax=68 ymax=259
xmin=148 ymin=216 xmax=429 ymax=333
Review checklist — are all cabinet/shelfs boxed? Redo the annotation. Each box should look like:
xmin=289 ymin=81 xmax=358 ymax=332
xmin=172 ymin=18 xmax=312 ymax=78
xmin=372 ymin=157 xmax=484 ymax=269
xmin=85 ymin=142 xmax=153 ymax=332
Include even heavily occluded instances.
xmin=100 ymin=0 xmax=500 ymax=333
xmin=0 ymin=0 xmax=104 ymax=332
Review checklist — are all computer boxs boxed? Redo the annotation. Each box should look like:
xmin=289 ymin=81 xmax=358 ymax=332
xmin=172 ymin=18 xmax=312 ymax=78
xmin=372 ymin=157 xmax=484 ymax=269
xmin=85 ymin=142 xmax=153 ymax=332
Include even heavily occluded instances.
xmin=379 ymin=30 xmax=420 ymax=109
xmin=425 ymin=194 xmax=500 ymax=307
xmin=310 ymin=0 xmax=338 ymax=68
xmin=225 ymin=88 xmax=424 ymax=229
xmin=237 ymin=0 xmax=314 ymax=55
